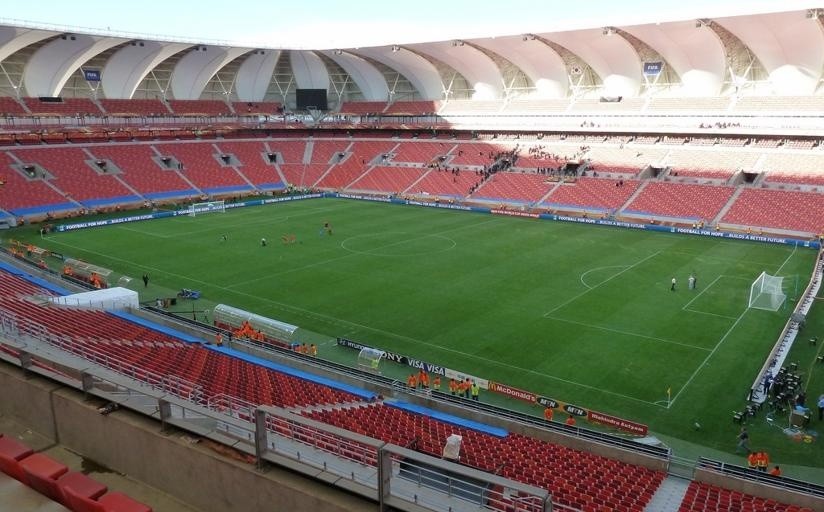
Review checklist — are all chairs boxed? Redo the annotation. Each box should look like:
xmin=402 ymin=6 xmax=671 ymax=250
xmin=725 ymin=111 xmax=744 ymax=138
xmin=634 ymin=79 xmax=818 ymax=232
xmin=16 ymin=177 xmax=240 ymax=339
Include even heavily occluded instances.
xmin=378 ymin=405 xmax=667 ymax=510
xmin=0 ymin=431 xmax=153 ymax=512
xmin=676 ymin=480 xmax=818 ymax=510
xmin=0 ymin=94 xmax=277 ymax=220
xmin=487 ymin=141 xmax=824 ymax=236
xmin=145 ymin=320 xmax=378 ymax=464
xmin=0 ymin=263 xmax=144 ymax=383
xmin=280 ymin=100 xmax=486 ymax=200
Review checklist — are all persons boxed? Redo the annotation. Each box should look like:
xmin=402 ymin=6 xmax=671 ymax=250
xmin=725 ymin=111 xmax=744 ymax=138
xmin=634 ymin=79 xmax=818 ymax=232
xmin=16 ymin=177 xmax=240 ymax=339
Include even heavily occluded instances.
xmin=421 ymin=145 xmax=590 ymax=196
xmin=142 ymin=271 xmax=149 ymax=289
xmin=544 ymin=405 xmax=553 ymax=419
xmin=40 ymin=222 xmax=50 ymax=236
xmin=215 ymin=319 xmax=264 ymax=348
xmin=36 ymin=259 xmax=50 ymax=271
xmin=17 ymin=217 xmax=26 ymax=229
xmin=141 ymin=198 xmax=157 ymax=213
xmin=408 ymin=366 xmax=480 ymax=402
xmin=669 ymin=276 xmax=677 ymax=291
xmin=9 ymin=243 xmax=33 ymax=261
xmin=735 ymin=335 xmax=824 ymax=475
xmin=687 ymin=272 xmax=696 ymax=292
xmin=567 ymin=413 xmax=577 ymax=425
xmin=294 ymin=340 xmax=318 ymax=356
xmin=62 ymin=265 xmax=76 ymax=276
xmin=89 ymin=271 xmax=101 ymax=289
xmin=154 ymin=297 xmax=169 ymax=310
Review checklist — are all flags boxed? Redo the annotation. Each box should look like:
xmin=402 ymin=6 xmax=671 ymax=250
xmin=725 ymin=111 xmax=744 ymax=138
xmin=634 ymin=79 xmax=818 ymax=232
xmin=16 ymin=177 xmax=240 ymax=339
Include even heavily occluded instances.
xmin=667 ymin=387 xmax=673 ymax=395
